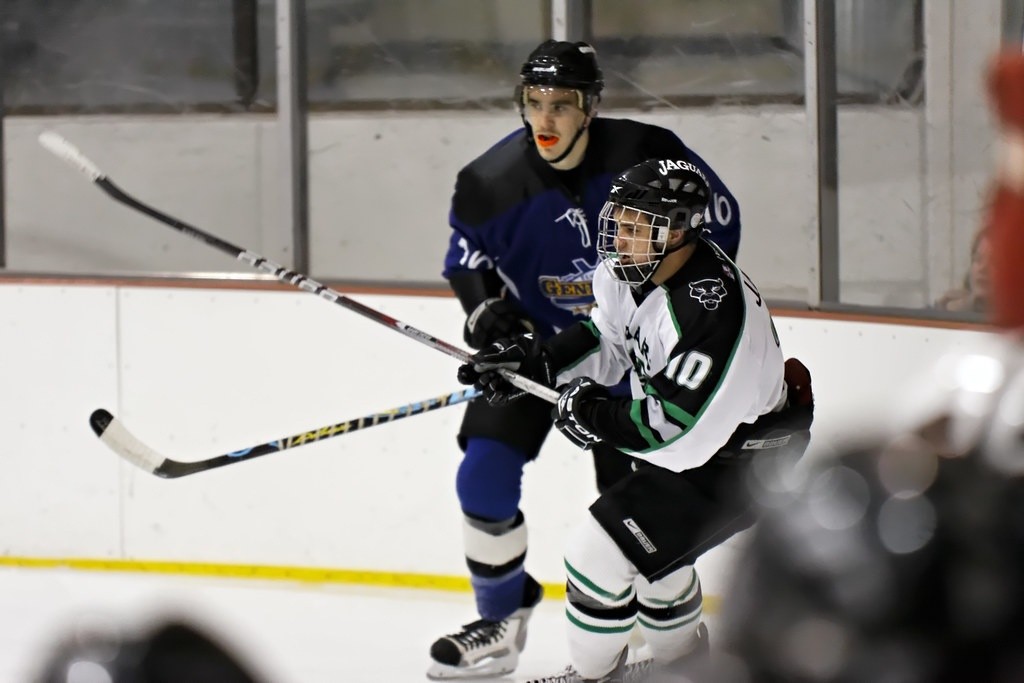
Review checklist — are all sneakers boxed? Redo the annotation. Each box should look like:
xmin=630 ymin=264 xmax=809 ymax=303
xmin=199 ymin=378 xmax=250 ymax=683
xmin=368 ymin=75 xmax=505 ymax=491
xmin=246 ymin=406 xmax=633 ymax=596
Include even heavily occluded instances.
xmin=427 ymin=574 xmax=544 ymax=680
xmin=528 ymin=644 xmax=628 ymax=683
xmin=624 ymin=621 xmax=709 ymax=681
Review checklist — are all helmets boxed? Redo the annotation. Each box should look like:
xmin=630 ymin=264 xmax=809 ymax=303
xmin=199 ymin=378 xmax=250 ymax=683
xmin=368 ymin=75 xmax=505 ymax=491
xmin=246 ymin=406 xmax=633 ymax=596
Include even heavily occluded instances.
xmin=520 ymin=40 xmax=606 ymax=93
xmin=596 ymin=158 xmax=716 ymax=288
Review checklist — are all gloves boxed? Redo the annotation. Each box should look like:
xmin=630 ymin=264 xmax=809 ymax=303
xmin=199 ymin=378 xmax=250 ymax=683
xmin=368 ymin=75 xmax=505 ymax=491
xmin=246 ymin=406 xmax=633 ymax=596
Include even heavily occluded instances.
xmin=549 ymin=375 xmax=616 ymax=449
xmin=458 ymin=337 xmax=533 ymax=397
xmin=463 ymin=297 xmax=537 ymax=350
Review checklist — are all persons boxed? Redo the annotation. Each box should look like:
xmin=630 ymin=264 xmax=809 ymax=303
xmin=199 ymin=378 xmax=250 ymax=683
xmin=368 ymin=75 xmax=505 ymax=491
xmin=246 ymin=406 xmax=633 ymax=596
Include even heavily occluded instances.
xmin=431 ymin=47 xmax=739 ymax=681
xmin=457 ymin=159 xmax=789 ymax=683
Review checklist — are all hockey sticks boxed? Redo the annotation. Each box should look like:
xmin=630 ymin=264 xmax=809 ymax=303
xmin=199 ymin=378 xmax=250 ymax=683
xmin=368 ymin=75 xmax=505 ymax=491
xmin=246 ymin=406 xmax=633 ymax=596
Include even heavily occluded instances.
xmin=91 ymin=387 xmax=484 ymax=480
xmin=37 ymin=132 xmax=565 ymax=402
